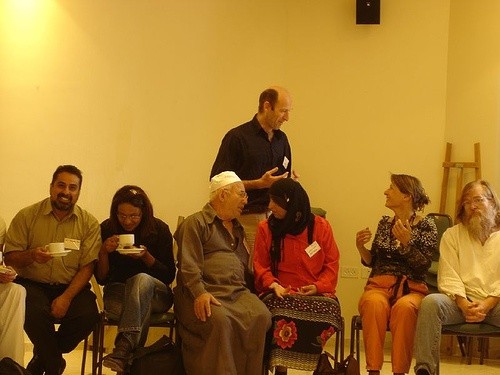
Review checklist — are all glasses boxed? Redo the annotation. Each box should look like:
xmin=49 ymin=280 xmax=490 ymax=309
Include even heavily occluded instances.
xmin=461 ymin=196 xmax=490 ymax=209
xmin=229 ymin=191 xmax=248 ymax=202
xmin=117 ymin=212 xmax=143 ymax=220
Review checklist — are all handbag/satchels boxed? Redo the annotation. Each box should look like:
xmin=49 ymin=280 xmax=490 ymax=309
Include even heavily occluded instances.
xmin=115 ymin=335 xmax=183 ymax=375
xmin=313 ymin=353 xmax=360 ymax=375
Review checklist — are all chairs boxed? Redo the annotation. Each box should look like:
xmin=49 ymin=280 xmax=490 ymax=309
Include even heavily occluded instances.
xmin=81 ymin=208 xmax=344 ymax=375
xmin=350 ymin=213 xmax=500 ymax=375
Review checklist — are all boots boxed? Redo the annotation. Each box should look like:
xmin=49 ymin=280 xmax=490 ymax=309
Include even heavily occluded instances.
xmin=103 ymin=332 xmax=135 ymax=372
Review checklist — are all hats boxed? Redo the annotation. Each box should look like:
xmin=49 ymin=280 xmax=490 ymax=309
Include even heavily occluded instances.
xmin=210 ymin=171 xmax=241 ymax=192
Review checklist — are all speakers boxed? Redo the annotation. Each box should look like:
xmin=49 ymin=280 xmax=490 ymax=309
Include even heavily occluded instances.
xmin=0 ymin=356 xmax=26 ymax=375
xmin=356 ymin=0 xmax=381 ymax=24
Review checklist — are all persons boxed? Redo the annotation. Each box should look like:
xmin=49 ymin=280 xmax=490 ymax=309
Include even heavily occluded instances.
xmin=0 ymin=216 xmax=34 ymax=375
xmin=253 ymin=178 xmax=340 ymax=375
xmin=209 ymin=86 xmax=299 ymax=254
xmin=355 ymin=174 xmax=438 ymax=375
xmin=5 ymin=166 xmax=101 ymax=375
xmin=174 ymin=171 xmax=273 ymax=375
xmin=94 ymin=184 xmax=176 ymax=375
xmin=414 ymin=180 xmax=500 ymax=375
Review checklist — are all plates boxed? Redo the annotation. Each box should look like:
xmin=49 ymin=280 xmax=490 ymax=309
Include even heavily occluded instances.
xmin=116 ymin=248 xmax=144 ymax=254
xmin=0 ymin=266 xmax=12 ymax=273
xmin=43 ymin=250 xmax=71 ymax=257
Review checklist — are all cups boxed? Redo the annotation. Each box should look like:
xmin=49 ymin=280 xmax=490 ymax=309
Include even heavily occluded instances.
xmin=115 ymin=234 xmax=135 ymax=246
xmin=45 ymin=242 xmax=66 ymax=252
xmin=0 ymin=251 xmax=2 ymax=265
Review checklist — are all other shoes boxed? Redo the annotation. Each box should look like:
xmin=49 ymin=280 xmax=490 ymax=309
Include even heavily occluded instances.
xmin=0 ymin=356 xmax=30 ymax=375
xmin=27 ymin=353 xmax=45 ymax=375
xmin=44 ymin=357 xmax=66 ymax=375
